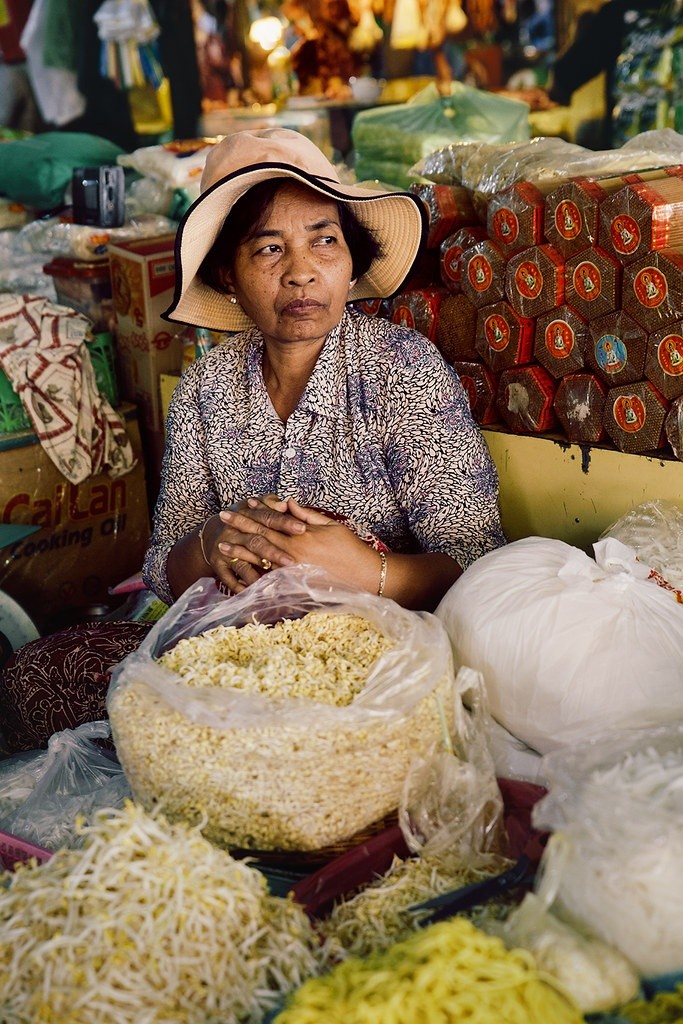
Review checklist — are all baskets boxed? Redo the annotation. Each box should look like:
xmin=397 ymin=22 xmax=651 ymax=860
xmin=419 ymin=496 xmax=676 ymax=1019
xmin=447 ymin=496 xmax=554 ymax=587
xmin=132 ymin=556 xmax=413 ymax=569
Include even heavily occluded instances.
xmin=0 ymin=778 xmax=683 ymax=1024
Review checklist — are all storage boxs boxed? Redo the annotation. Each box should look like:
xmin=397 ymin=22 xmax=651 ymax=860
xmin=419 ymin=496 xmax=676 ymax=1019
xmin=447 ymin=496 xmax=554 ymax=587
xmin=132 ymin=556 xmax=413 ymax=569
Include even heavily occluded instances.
xmin=108 ymin=231 xmax=195 ymax=432
xmin=0 ymin=332 xmax=119 ymax=436
xmin=0 ymin=400 xmax=152 ymax=658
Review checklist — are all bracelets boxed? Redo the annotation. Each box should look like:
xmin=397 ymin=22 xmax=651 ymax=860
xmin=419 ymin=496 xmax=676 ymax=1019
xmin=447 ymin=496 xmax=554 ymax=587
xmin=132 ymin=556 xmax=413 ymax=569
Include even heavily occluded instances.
xmin=198 ymin=513 xmax=219 ymax=565
xmin=377 ymin=551 xmax=387 ymax=598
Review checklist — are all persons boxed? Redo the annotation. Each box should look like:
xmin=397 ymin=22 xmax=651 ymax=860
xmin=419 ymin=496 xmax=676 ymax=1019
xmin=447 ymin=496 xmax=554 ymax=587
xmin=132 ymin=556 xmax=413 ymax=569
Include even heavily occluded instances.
xmin=141 ymin=128 xmax=511 ymax=626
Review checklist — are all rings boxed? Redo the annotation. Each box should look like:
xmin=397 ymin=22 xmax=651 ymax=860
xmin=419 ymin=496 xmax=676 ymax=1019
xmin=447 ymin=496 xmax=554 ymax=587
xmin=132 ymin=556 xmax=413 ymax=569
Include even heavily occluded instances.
xmin=228 ymin=557 xmax=238 ymax=569
xmin=261 ymin=557 xmax=272 ymax=569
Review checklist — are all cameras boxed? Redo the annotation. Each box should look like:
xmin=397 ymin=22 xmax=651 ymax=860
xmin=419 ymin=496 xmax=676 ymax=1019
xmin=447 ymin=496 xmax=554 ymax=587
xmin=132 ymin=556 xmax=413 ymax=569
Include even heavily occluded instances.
xmin=72 ymin=164 xmax=127 ymax=228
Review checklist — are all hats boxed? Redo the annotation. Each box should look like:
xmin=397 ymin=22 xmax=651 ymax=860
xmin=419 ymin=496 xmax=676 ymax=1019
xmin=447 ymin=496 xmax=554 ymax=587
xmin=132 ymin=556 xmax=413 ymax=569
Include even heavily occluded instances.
xmin=160 ymin=128 xmax=430 ymax=335
